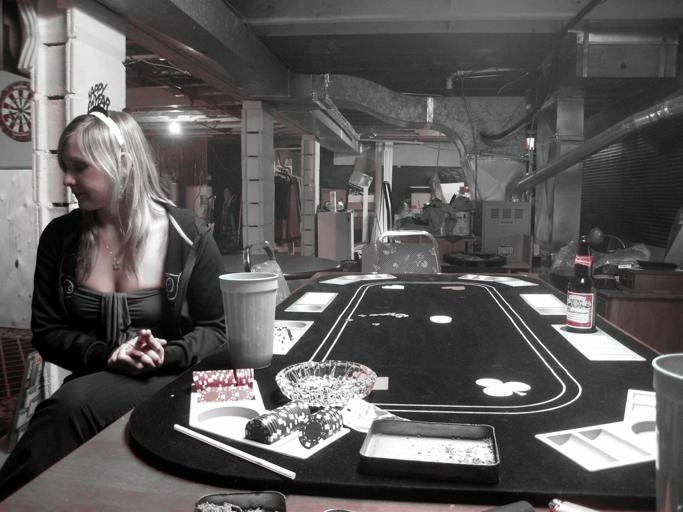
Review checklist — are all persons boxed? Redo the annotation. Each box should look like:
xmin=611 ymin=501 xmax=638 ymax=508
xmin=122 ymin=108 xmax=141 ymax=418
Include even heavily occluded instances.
xmin=0 ymin=106 xmax=227 ymax=500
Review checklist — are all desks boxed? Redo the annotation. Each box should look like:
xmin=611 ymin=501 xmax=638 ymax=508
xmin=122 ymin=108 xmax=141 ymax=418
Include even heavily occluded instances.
xmin=4 ymin=260 xmax=679 ymax=509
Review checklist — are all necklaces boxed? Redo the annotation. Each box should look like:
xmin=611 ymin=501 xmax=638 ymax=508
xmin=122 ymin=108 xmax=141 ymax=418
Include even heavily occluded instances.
xmin=101 ymin=239 xmax=122 ymax=270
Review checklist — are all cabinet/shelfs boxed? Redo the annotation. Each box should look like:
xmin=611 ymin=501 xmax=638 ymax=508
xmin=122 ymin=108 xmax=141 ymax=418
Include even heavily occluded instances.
xmin=316 ymin=186 xmax=533 ymax=276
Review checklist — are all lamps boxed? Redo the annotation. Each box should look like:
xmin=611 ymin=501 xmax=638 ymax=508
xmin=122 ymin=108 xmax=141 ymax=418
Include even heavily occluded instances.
xmin=158 ymin=113 xmax=189 ymax=141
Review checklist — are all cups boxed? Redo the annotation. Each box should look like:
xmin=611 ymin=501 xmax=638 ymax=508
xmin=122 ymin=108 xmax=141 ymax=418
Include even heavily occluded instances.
xmin=218 ymin=270 xmax=279 ymax=371
xmin=651 ymin=351 xmax=683 ymax=512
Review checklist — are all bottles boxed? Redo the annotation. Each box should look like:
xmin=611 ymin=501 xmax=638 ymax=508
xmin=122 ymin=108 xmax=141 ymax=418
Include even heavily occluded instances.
xmin=563 ymin=234 xmax=599 ymax=334
